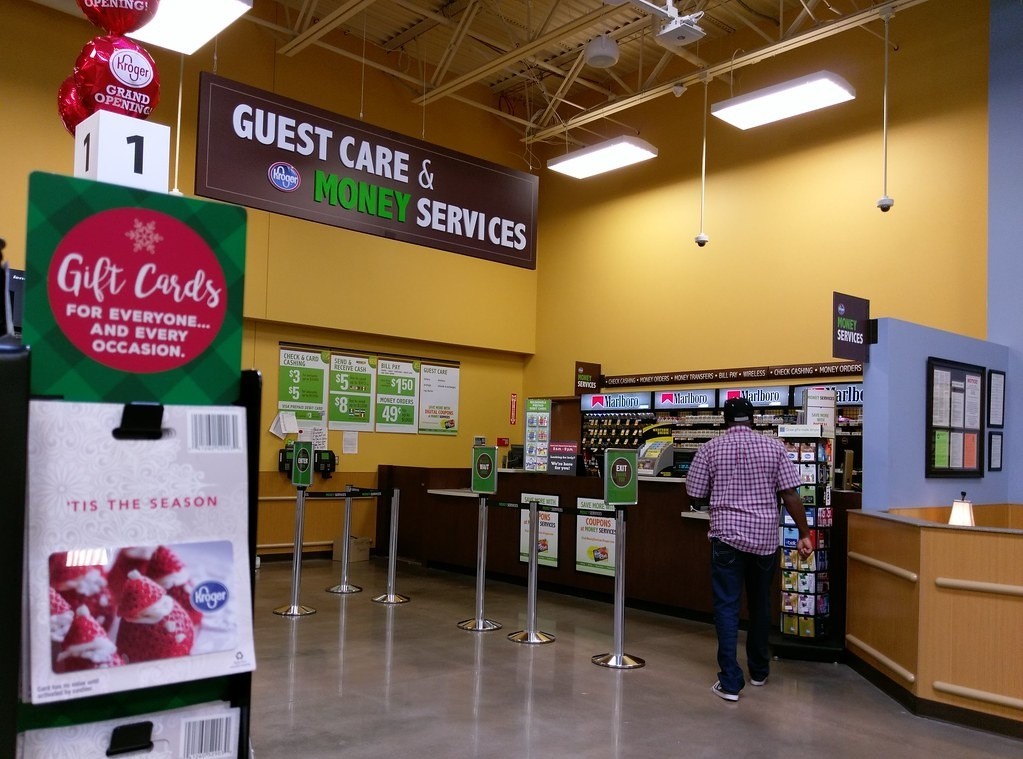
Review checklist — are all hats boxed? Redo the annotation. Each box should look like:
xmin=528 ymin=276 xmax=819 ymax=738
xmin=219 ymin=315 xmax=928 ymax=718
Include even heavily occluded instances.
xmin=724 ymin=398 xmax=754 ymax=423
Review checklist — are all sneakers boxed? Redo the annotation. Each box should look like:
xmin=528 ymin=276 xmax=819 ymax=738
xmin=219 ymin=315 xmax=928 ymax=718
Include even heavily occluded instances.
xmin=712 ymin=680 xmax=739 ymax=700
xmin=750 ymin=676 xmax=768 ymax=685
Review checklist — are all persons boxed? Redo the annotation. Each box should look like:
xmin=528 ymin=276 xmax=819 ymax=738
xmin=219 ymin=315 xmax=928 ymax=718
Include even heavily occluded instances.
xmin=686 ymin=397 xmax=813 ymax=702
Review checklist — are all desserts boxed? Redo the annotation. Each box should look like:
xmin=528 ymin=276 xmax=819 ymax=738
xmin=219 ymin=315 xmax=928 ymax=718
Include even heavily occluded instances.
xmin=48 ymin=545 xmax=204 ymax=674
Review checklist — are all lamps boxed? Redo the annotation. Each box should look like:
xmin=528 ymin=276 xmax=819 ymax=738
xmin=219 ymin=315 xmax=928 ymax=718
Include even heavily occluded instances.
xmin=709 ymin=51 xmax=856 ymax=130
xmin=122 ymin=1 xmax=256 ymax=56
xmin=545 ymin=127 xmax=658 ymax=179
